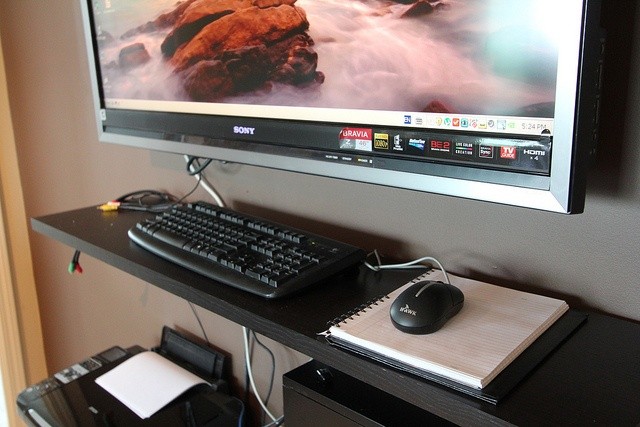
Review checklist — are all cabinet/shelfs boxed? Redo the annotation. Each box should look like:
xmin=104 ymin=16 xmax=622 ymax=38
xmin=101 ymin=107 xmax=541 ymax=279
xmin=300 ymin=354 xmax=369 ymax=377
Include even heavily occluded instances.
xmin=283 ymin=359 xmax=459 ymax=427
xmin=30 ymin=196 xmax=640 ymax=427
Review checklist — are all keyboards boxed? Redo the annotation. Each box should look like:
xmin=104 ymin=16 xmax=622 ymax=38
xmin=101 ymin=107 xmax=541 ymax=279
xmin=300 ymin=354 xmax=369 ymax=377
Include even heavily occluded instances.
xmin=127 ymin=200 xmax=366 ymax=298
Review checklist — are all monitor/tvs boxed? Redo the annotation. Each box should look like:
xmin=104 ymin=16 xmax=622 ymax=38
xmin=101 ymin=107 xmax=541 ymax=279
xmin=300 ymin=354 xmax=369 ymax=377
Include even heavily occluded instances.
xmin=80 ymin=0 xmax=598 ymax=214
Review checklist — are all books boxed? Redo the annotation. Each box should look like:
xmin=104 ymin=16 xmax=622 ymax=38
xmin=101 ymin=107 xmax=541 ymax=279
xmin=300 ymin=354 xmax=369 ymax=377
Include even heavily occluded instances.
xmin=316 ymin=266 xmax=590 ymax=407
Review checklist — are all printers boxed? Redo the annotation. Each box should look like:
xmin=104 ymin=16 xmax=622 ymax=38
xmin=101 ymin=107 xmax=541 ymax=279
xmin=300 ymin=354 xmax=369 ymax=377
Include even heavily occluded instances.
xmin=16 ymin=325 xmax=252 ymax=427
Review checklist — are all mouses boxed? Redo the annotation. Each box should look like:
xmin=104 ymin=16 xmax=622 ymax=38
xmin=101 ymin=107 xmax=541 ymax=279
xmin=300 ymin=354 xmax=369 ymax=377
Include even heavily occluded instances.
xmin=390 ymin=280 xmax=464 ymax=335
